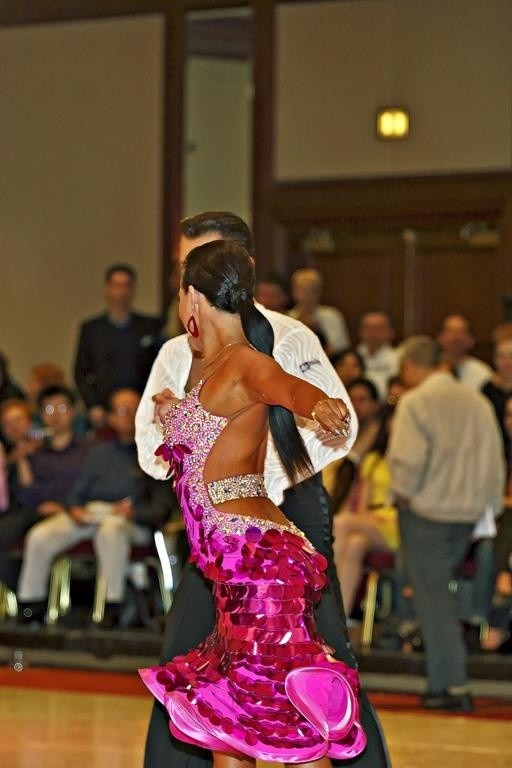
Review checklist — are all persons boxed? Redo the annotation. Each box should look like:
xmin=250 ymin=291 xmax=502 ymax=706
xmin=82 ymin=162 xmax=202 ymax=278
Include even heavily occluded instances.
xmin=133 ymin=209 xmax=393 ymax=768
xmin=0 ymin=263 xmax=188 ymax=631
xmin=261 ymin=270 xmax=512 ymax=712
xmin=152 ymin=241 xmax=349 ymax=768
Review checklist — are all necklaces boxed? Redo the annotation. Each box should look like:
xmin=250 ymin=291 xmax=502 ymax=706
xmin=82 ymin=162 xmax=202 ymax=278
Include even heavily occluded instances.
xmin=201 ymin=339 xmax=244 ymax=368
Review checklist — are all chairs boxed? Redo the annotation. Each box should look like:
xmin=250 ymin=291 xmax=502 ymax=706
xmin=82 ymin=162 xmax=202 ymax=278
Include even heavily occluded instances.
xmin=3 ymin=519 xmax=511 ymax=656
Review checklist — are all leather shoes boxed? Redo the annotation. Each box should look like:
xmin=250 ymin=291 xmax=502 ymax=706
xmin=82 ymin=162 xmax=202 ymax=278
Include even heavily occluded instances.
xmin=420 ymin=691 xmax=474 ymax=712
